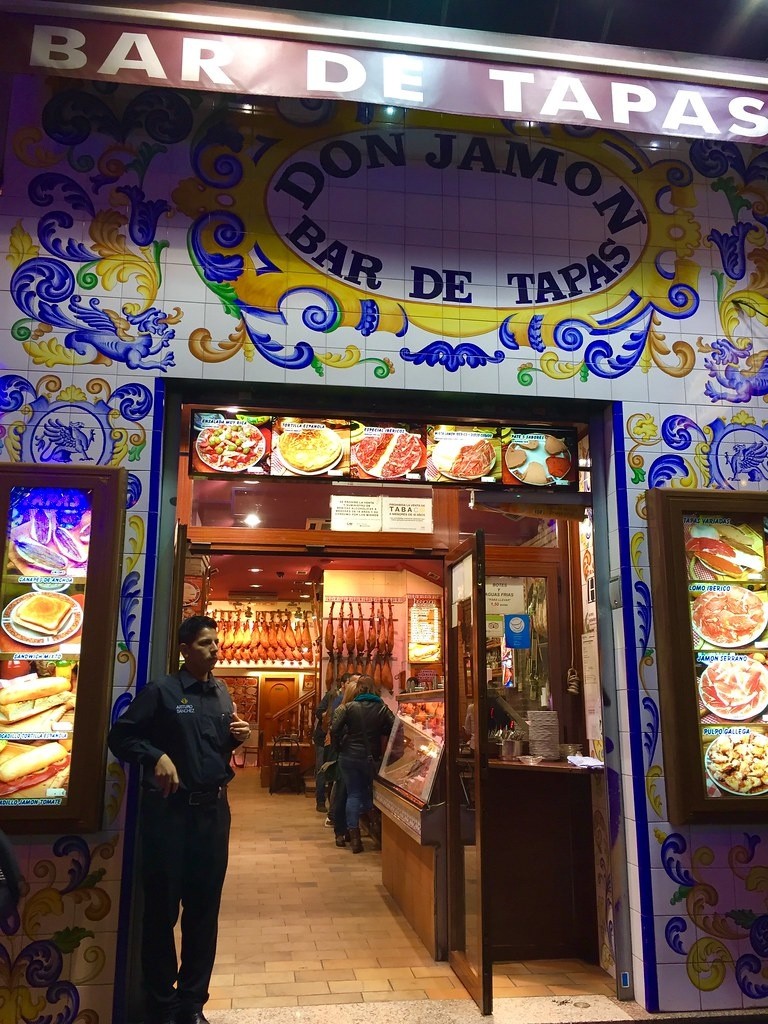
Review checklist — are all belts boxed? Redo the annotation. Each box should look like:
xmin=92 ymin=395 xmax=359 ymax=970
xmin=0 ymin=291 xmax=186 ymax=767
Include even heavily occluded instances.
xmin=143 ymin=786 xmax=227 ymax=806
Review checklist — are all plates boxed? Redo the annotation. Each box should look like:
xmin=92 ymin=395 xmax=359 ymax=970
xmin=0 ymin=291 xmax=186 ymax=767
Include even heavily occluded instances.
xmin=195 ymin=419 xmax=266 ymax=472
xmin=1 ymin=591 xmax=83 ymax=646
xmin=692 ymin=586 xmax=768 ymax=647
xmin=278 ymin=427 xmax=344 ymax=476
xmin=180 ymin=581 xmax=199 ymax=607
xmin=357 ymin=430 xmax=422 ymax=478
xmin=699 ymin=656 xmax=768 ymax=720
xmin=704 ymin=732 xmax=768 ymax=796
xmin=506 ymin=433 xmax=572 ymax=485
xmin=700 ymin=524 xmax=753 ymax=575
xmin=432 ymin=432 xmax=497 ymax=482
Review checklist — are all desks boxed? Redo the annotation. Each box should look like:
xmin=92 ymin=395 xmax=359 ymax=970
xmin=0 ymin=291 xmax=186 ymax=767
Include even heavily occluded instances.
xmin=266 ymin=741 xmax=310 ymax=792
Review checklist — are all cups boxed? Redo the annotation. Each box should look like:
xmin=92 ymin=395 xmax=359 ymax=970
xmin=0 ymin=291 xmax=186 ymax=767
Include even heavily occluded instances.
xmin=502 ymin=740 xmax=521 ymax=761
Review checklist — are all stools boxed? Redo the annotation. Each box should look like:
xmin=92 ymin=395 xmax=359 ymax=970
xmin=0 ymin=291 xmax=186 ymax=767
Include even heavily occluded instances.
xmin=242 ymin=746 xmax=260 ymax=767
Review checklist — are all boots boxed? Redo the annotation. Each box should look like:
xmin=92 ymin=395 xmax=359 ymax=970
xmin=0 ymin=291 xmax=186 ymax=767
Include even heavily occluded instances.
xmin=370 ymin=825 xmax=382 ymax=851
xmin=348 ymin=828 xmax=365 ymax=854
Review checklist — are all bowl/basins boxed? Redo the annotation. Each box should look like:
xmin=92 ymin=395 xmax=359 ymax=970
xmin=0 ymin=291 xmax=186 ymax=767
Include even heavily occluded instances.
xmin=518 ymin=756 xmax=543 ymax=764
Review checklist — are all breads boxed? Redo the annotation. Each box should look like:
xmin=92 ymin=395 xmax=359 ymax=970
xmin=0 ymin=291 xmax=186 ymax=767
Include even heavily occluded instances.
xmin=279 ymin=428 xmax=341 ymax=471
xmin=0 ymin=677 xmax=72 ymax=725
xmin=0 ymin=742 xmax=68 ymax=782
xmin=13 ymin=595 xmax=71 ymax=635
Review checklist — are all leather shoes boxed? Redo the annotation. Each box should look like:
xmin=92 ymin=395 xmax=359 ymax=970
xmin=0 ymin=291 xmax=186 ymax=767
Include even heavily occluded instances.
xmin=190 ymin=1011 xmax=215 ymax=1024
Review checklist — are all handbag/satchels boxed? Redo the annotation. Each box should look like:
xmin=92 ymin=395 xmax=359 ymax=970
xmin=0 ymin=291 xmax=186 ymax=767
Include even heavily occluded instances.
xmin=366 ymin=754 xmax=383 ymax=773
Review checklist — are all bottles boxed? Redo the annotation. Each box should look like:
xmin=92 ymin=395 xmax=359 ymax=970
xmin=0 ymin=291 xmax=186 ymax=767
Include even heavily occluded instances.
xmin=487 ymin=650 xmax=500 ymax=668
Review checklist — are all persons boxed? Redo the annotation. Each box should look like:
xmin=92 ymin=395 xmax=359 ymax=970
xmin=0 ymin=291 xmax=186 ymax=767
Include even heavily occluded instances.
xmin=399 ymin=677 xmax=419 ymax=693
xmin=105 ymin=615 xmax=253 ymax=1024
xmin=315 ymin=672 xmax=350 ymax=812
xmin=330 ymin=674 xmax=403 ymax=853
xmin=463 ymin=702 xmax=477 ymax=758
xmin=327 ymin=674 xmax=362 ymax=846
xmin=321 ymin=674 xmax=363 ymax=827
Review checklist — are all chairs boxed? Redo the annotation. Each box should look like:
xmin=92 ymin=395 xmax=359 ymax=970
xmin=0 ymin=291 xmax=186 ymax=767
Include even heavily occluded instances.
xmin=269 ymin=738 xmax=301 ymax=795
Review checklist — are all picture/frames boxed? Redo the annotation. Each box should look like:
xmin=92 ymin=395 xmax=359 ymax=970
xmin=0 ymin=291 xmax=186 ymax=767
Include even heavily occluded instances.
xmin=302 ymin=674 xmax=315 ymax=690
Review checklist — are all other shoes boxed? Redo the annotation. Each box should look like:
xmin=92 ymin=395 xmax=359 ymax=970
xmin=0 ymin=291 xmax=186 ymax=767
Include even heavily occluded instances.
xmin=336 ymin=829 xmax=351 ymax=847
xmin=327 ymin=820 xmax=335 ymax=825
xmin=316 ymin=802 xmax=327 ymax=813
